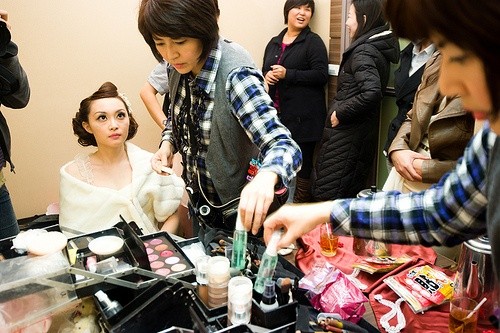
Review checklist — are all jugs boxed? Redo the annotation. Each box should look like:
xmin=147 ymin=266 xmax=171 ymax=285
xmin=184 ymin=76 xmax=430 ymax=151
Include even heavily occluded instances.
xmin=353 ymin=186 xmax=392 ymax=256
xmin=454 ymin=235 xmax=500 ymax=324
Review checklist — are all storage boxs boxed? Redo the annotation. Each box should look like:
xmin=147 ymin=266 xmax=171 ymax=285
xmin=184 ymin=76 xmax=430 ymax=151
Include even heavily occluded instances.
xmin=64 ymin=214 xmax=299 ymax=333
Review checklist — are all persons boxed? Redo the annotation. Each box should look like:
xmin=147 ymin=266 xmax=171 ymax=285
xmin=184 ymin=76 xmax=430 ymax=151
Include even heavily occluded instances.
xmin=138 ymin=0 xmax=304 ymax=237
xmin=262 ymin=0 xmax=500 ymax=292
xmin=263 ymin=0 xmax=329 ymax=207
xmin=311 ymin=1 xmax=401 ymax=203
xmin=140 ymin=0 xmax=270 ymax=130
xmin=383 ymin=33 xmax=477 ymax=264
xmin=57 ymin=80 xmax=186 ymax=240
xmin=0 ymin=7 xmax=29 ymax=260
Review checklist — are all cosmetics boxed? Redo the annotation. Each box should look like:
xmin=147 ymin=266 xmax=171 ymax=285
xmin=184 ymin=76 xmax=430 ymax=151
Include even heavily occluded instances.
xmin=313 ymin=305 xmax=369 ymax=333
xmin=0 ymin=210 xmax=455 ymax=333
xmin=317 ymin=218 xmax=339 ymax=259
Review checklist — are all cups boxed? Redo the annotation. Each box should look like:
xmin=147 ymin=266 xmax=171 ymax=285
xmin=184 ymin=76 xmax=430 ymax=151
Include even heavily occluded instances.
xmin=320 ymin=222 xmax=338 ymax=257
xmin=448 ymin=297 xmax=479 ymax=333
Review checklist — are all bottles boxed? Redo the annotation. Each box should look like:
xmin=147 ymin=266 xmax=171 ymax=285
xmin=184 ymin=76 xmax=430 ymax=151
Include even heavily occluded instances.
xmin=253 ymin=231 xmax=282 ymax=294
xmin=231 ymin=212 xmax=247 ymax=270
xmin=260 ymin=281 xmax=279 ymax=312
